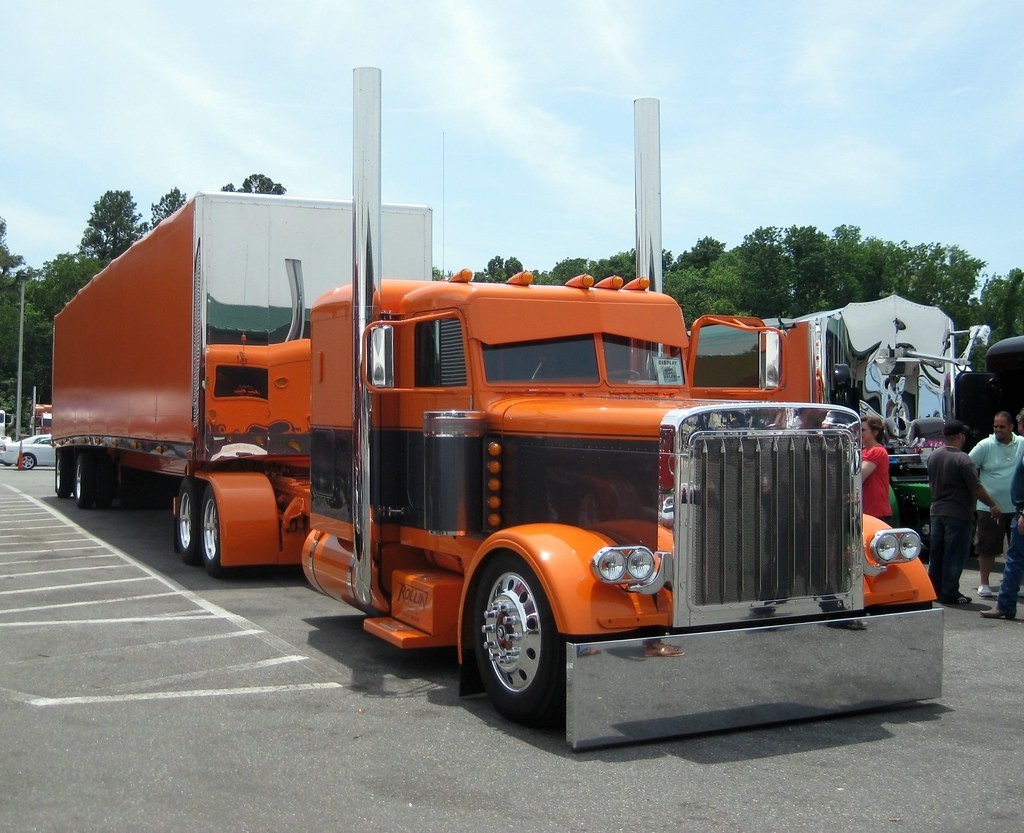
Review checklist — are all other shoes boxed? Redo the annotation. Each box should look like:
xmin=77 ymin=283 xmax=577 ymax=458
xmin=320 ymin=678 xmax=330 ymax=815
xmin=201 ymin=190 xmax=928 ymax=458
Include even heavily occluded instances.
xmin=578 ymin=646 xmax=602 ymax=656
xmin=938 ymin=596 xmax=972 ymax=604
xmin=977 ymin=584 xmax=993 ymax=596
xmin=979 ymin=607 xmax=1016 ymax=620
xmin=1017 ymin=585 xmax=1024 ymax=596
xmin=643 ymin=643 xmax=685 ymax=657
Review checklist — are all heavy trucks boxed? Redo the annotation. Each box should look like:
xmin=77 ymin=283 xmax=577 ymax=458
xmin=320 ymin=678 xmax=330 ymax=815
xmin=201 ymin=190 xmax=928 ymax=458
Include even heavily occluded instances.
xmin=51 ymin=67 xmax=944 ymax=749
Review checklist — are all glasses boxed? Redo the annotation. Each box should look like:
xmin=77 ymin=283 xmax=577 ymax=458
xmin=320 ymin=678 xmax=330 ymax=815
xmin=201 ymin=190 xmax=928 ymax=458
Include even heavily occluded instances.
xmin=862 ymin=429 xmax=872 ymax=431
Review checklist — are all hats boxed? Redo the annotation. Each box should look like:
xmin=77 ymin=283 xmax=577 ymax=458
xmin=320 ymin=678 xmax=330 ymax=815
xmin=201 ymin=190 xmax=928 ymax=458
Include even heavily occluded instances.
xmin=943 ymin=420 xmax=970 ymax=435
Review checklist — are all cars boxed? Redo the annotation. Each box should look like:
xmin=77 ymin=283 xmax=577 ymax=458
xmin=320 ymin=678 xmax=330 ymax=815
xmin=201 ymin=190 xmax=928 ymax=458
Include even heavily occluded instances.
xmin=0 ymin=434 xmax=62 ymax=470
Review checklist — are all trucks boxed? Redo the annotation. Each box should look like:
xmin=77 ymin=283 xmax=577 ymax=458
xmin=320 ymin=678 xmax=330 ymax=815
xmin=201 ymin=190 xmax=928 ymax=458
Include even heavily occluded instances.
xmin=30 ymin=404 xmax=53 ymax=434
xmin=684 ymin=294 xmax=1024 ymax=559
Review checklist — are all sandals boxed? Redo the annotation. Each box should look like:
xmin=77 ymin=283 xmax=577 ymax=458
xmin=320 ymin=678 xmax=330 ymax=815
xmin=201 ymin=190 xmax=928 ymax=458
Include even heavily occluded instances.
xmin=829 ymin=620 xmax=867 ymax=628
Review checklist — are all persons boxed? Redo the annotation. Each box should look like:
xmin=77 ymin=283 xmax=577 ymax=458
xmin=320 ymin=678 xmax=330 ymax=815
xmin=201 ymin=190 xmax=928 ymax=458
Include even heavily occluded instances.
xmin=968 ymin=412 xmax=1024 ymax=596
xmin=860 ymin=415 xmax=892 ymax=527
xmin=979 ymin=409 xmax=1024 ymax=619
xmin=926 ymin=420 xmax=1001 ymax=603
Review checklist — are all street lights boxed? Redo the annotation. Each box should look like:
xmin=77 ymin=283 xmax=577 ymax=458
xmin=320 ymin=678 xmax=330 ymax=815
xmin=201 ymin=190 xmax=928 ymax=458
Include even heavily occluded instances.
xmin=16 ymin=274 xmax=29 ymax=441
